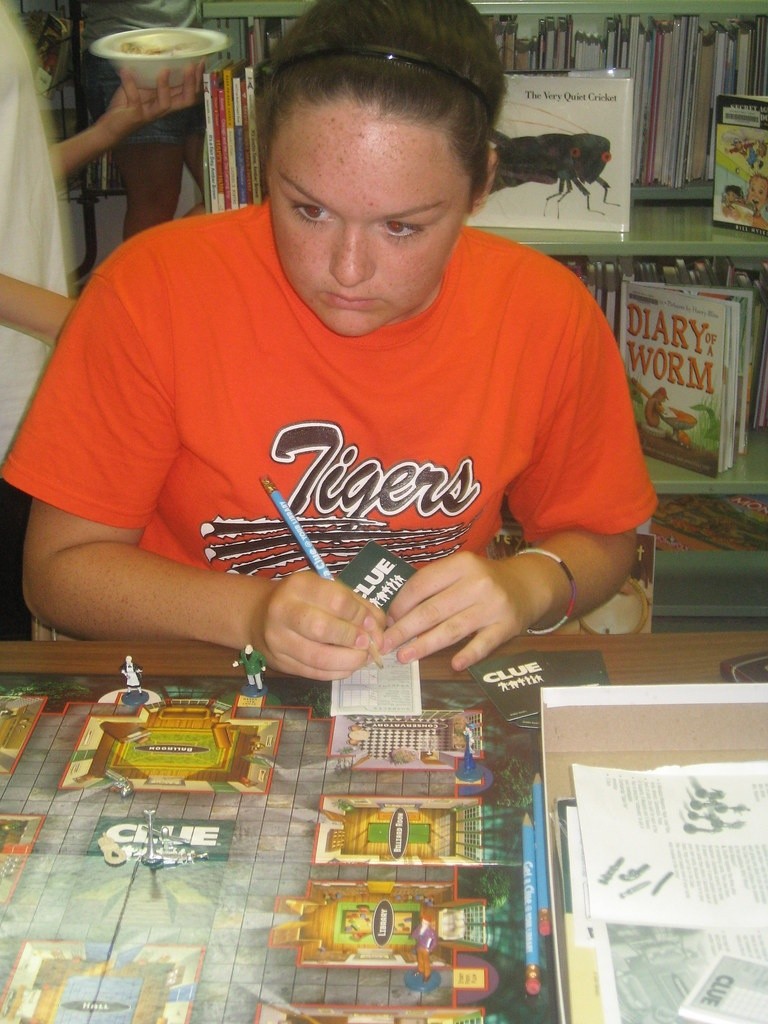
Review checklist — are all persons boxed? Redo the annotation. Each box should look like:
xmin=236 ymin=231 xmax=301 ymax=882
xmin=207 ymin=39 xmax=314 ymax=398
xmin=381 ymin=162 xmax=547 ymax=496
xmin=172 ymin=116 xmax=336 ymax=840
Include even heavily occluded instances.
xmin=120 ymin=656 xmax=144 ymax=696
xmin=232 ymin=645 xmax=267 ymax=692
xmin=463 ymin=723 xmax=476 ymax=773
xmin=411 ymin=916 xmax=436 ymax=983
xmin=1 ymin=0 xmax=204 ymax=644
xmin=0 ymin=0 xmax=658 ymax=684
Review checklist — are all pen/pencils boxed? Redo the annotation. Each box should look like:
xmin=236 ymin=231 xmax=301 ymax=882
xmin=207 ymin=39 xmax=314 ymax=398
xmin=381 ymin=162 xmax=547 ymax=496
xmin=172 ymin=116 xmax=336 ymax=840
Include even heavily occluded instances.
xmin=261 ymin=471 xmax=383 ymax=671
xmin=532 ymin=773 xmax=551 ymax=937
xmin=522 ymin=811 xmax=541 ymax=996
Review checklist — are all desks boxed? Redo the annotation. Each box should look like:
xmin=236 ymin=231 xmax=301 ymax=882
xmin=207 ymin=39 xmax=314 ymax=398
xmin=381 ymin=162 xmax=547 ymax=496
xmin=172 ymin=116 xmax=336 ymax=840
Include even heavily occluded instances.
xmin=0 ymin=634 xmax=768 ymax=1024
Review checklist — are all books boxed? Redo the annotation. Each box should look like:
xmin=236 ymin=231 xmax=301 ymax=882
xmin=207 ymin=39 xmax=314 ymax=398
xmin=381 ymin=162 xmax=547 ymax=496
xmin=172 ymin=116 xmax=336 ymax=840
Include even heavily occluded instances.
xmin=557 ymin=255 xmax=768 ymax=478
xmin=490 ymin=508 xmax=657 ymax=635
xmin=203 ymin=14 xmax=768 ymax=237
xmin=555 ymin=796 xmax=601 ymax=1024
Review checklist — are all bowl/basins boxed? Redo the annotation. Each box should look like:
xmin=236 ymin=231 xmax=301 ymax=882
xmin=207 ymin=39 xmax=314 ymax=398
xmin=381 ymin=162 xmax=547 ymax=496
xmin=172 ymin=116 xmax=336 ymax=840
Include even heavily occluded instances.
xmin=87 ymin=26 xmax=235 ymax=90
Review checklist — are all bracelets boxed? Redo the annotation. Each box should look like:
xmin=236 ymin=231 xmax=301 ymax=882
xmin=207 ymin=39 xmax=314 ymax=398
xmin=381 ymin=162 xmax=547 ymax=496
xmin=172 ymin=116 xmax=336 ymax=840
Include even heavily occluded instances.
xmin=516 ymin=549 xmax=577 ymax=636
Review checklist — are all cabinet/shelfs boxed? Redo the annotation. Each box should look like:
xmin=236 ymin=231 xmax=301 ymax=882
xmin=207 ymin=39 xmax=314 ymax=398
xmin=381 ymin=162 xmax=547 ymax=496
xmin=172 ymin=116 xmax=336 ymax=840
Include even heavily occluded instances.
xmin=200 ymin=0 xmax=768 ymax=622
